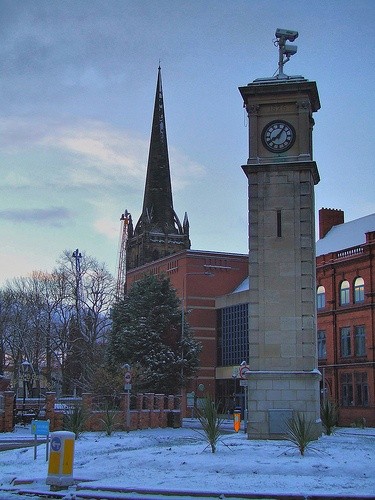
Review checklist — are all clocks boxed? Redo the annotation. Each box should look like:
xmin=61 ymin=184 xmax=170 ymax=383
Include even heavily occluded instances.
xmin=261 ymin=119 xmax=296 ymax=153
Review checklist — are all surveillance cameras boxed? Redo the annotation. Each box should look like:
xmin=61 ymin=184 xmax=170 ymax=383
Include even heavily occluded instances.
xmin=275 ymin=28 xmax=299 ymax=42
xmin=281 ymin=45 xmax=297 ymax=55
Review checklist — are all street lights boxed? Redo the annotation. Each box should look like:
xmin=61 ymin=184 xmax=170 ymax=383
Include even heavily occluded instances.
xmin=21 ymin=361 xmax=31 ymax=426
xmin=178 ymin=273 xmax=215 ymax=413
